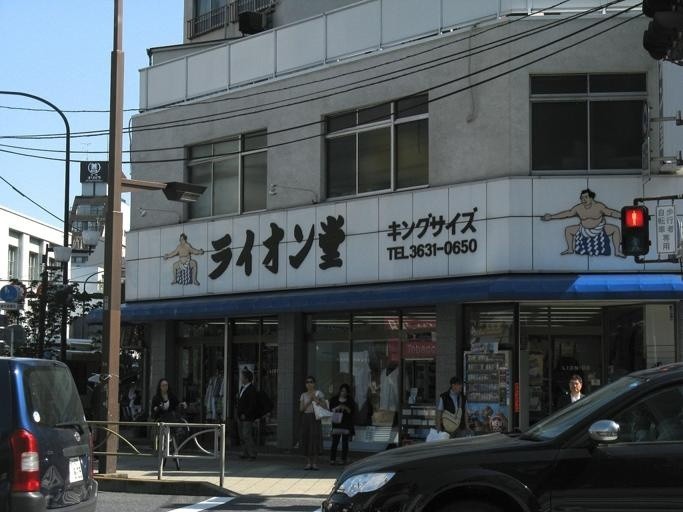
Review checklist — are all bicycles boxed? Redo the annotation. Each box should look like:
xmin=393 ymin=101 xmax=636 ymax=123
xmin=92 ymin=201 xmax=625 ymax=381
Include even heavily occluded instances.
xmin=155 ymin=401 xmax=187 ymax=471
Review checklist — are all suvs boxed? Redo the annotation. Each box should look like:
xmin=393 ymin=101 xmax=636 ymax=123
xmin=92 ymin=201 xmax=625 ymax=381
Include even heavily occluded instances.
xmin=321 ymin=362 xmax=682 ymax=512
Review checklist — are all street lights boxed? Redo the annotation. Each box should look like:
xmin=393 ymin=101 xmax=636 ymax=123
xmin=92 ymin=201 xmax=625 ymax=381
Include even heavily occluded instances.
xmin=96 ymin=179 xmax=207 ymax=485
xmin=52 ymin=230 xmax=99 ymax=365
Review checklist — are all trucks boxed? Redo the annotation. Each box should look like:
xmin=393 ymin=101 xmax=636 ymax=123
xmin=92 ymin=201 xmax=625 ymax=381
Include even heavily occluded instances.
xmin=47 ymin=348 xmax=139 ymax=411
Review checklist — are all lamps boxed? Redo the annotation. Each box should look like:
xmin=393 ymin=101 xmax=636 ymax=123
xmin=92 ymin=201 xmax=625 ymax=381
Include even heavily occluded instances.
xmin=134 ymin=324 xmax=144 ymax=336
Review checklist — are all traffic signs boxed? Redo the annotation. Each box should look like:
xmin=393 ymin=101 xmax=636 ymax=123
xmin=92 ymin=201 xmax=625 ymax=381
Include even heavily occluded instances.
xmin=0 ymin=301 xmax=19 ymax=311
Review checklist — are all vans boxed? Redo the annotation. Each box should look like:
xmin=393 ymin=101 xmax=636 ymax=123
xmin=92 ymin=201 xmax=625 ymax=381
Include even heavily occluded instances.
xmin=0 ymin=356 xmax=98 ymax=512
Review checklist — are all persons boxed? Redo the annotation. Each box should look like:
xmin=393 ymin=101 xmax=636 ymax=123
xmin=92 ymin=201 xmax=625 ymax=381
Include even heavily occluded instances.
xmin=557 ymin=375 xmax=586 ymax=411
xmin=540 ymin=189 xmax=625 ymax=258
xmin=379 ymin=360 xmax=400 ymax=412
xmin=235 ymin=372 xmax=259 ymax=461
xmin=153 ymin=378 xmax=187 ymax=469
xmin=329 ymin=383 xmax=360 ymax=466
xmin=162 ymin=233 xmax=202 ymax=285
xmin=299 ymin=375 xmax=327 ymax=469
xmin=436 ymin=375 xmax=475 ymax=439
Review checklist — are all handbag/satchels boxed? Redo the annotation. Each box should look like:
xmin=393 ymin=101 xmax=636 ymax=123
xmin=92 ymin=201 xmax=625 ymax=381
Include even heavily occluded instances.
xmin=248 ymin=390 xmax=274 ymax=420
xmin=332 ymin=408 xmax=343 ymax=423
xmin=312 ymin=400 xmax=332 ymax=421
xmin=442 ymin=407 xmax=462 ymax=433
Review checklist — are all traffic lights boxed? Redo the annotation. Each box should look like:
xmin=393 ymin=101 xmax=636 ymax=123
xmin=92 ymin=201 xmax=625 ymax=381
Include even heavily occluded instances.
xmin=620 ymin=206 xmax=649 ymax=255
xmin=641 ymin=2 xmax=673 ymax=16
xmin=642 ymin=11 xmax=679 ymax=61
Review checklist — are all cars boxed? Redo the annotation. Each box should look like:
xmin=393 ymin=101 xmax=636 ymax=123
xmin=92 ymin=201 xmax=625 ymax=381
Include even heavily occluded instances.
xmin=52 ymin=338 xmax=95 ymax=351
